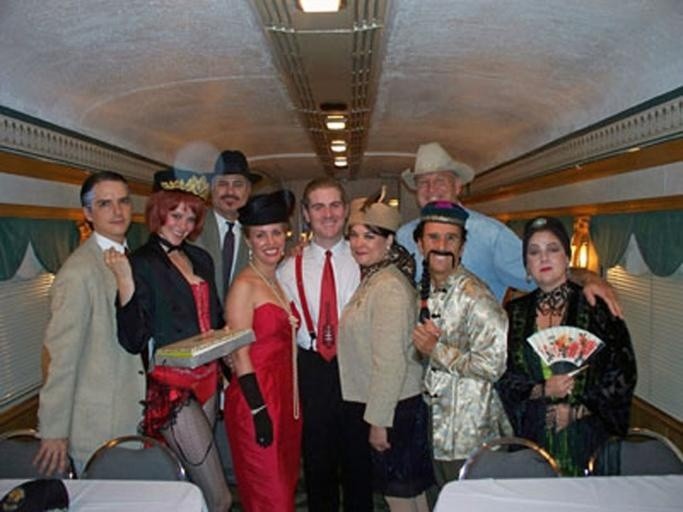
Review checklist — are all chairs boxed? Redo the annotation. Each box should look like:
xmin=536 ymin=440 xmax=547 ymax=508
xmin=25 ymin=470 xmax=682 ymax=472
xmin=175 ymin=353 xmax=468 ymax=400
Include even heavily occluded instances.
xmin=458 ymin=436 xmax=562 ymax=476
xmin=589 ymin=425 xmax=682 ymax=475
xmin=0 ymin=428 xmax=76 ymax=478
xmin=79 ymin=432 xmax=186 ymax=481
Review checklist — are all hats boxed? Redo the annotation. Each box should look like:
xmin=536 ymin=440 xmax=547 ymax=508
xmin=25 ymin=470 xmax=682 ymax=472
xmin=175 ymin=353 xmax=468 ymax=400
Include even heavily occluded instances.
xmin=155 ymin=151 xmax=296 ymax=224
xmin=523 ymin=214 xmax=566 ymax=237
xmin=344 ymin=142 xmax=475 ymax=235
xmin=1 ymin=480 xmax=68 ymax=512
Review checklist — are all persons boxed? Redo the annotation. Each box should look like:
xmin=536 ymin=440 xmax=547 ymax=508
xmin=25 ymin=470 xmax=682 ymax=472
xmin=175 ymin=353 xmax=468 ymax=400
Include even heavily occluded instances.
xmin=335 ymin=196 xmax=437 ymax=511
xmin=412 ymin=200 xmax=510 ymax=487
xmin=499 ymin=215 xmax=638 ymax=475
xmin=284 ymin=178 xmax=372 ymax=509
xmin=184 ymin=150 xmax=254 ymax=308
xmin=390 ymin=142 xmax=627 ymax=319
xmin=103 ymin=190 xmax=233 ymax=511
xmin=32 ymin=170 xmax=154 ymax=478
xmin=223 ymin=189 xmax=305 ymax=511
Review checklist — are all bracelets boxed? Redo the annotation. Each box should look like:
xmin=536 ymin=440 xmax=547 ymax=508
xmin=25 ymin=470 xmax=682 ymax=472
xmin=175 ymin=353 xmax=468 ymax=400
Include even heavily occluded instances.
xmin=569 ymin=403 xmax=588 ymax=419
xmin=249 ymin=404 xmax=268 ymax=415
xmin=539 ymin=379 xmax=549 ymax=402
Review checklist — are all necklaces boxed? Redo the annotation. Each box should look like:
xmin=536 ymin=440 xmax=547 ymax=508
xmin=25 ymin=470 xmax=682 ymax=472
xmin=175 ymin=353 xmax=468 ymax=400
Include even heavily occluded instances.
xmin=251 ymin=272 xmax=282 ymax=288
xmin=246 ymin=259 xmax=300 ymax=424
xmin=535 ymin=284 xmax=572 ymax=326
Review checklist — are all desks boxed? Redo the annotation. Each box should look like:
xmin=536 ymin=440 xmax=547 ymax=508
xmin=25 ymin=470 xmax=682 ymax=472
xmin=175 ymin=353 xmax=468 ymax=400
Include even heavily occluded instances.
xmin=0 ymin=475 xmax=210 ymax=512
xmin=430 ymin=478 xmax=682 ymax=510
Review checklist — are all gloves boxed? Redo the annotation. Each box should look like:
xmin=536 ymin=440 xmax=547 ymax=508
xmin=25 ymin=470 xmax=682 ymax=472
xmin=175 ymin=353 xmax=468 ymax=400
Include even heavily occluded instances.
xmin=238 ymin=373 xmax=273 ymax=447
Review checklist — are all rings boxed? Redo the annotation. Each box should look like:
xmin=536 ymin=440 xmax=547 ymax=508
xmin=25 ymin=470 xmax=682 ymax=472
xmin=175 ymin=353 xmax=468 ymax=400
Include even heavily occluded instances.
xmin=568 ymin=383 xmax=575 ymax=393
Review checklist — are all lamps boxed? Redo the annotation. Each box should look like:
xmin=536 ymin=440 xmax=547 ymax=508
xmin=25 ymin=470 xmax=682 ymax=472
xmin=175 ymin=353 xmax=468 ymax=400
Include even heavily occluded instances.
xmin=325 ymin=108 xmax=348 ymax=168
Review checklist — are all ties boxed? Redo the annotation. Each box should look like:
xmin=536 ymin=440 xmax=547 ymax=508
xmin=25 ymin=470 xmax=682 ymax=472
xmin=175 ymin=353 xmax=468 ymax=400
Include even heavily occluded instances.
xmin=317 ymin=251 xmax=338 ymax=362
xmin=222 ymin=221 xmax=234 ymax=300
